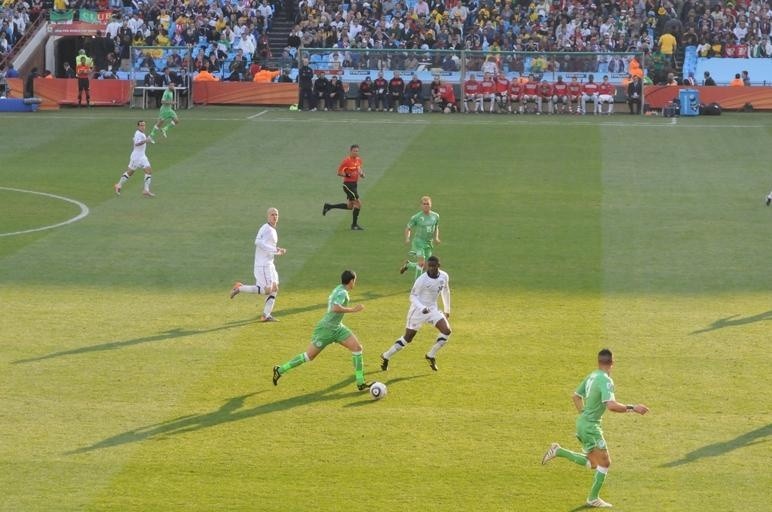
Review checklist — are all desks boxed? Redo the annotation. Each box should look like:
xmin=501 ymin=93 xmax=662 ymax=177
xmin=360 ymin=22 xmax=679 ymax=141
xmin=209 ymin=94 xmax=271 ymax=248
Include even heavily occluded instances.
xmin=134 ymin=86 xmax=187 ymax=111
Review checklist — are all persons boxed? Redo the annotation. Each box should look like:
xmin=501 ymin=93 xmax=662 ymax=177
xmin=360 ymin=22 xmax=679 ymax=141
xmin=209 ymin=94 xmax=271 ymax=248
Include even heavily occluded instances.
xmin=273 ymin=270 xmax=376 ymax=392
xmin=381 ymin=256 xmax=451 ymax=371
xmin=0 ymin=0 xmax=767 ymax=115
xmin=115 ymin=121 xmax=157 ymax=200
xmin=400 ymin=196 xmax=439 ymax=283
xmin=148 ymin=82 xmax=180 ymax=144
xmin=543 ymin=349 xmax=649 ymax=507
xmin=230 ymin=207 xmax=287 ymax=322
xmin=322 ymin=145 xmax=366 ymax=229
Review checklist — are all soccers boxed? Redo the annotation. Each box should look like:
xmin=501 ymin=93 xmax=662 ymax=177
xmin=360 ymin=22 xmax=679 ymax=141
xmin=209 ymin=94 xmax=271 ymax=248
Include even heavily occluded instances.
xmin=370 ymin=382 xmax=385 ymax=398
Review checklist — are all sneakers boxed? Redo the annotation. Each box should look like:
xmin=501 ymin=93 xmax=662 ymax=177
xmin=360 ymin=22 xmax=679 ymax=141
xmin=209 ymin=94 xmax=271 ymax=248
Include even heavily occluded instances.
xmin=272 ymin=366 xmax=279 ymax=385
xmin=585 ymin=497 xmax=612 ymax=507
xmin=380 ymin=353 xmax=388 ymax=371
xmin=142 ymin=191 xmax=154 ymax=199
xmin=323 ymin=203 xmax=329 ymax=215
xmin=229 ymin=282 xmax=241 ymax=299
xmin=114 ymin=184 xmax=122 ymax=196
xmin=260 ymin=315 xmax=278 ymax=322
xmin=401 ymin=260 xmax=409 ymax=273
xmin=425 ymin=354 xmax=437 ymax=371
xmin=356 ymin=108 xmax=393 ymax=112
xmin=148 ymin=129 xmax=167 ymax=142
xmin=310 ymin=107 xmax=328 ymax=111
xmin=352 ymin=224 xmax=363 ymax=230
xmin=357 ymin=381 xmax=375 ymax=390
xmin=542 ymin=443 xmax=559 ymax=465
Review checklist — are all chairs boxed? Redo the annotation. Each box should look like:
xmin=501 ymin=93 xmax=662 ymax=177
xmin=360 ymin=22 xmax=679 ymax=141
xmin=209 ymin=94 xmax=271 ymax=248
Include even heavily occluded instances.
xmin=422 ymin=83 xmax=431 ymax=100
xmin=614 ymin=87 xmax=626 ymax=103
xmin=453 ymin=84 xmax=460 ymax=100
xmin=345 ymin=82 xmax=358 ymax=100
